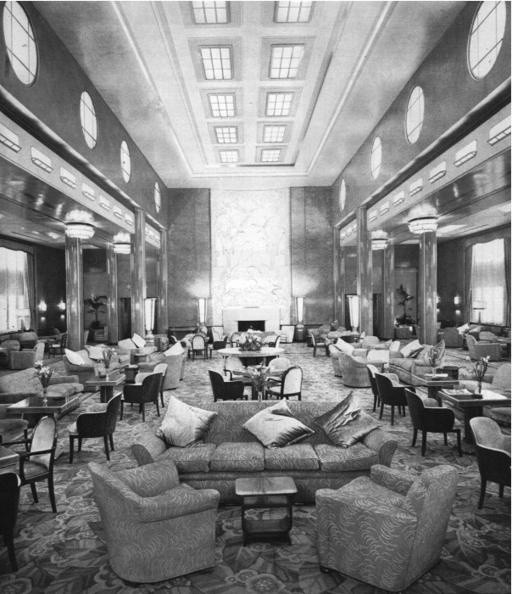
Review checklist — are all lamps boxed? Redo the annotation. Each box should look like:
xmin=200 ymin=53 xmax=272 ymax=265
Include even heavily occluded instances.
xmin=370 ymin=237 xmax=387 ymax=251
xmin=199 ymin=298 xmax=205 ymax=322
xmin=406 ymin=216 xmax=438 ymax=234
xmin=348 ymin=294 xmax=358 ymax=327
xmin=372 ymin=228 xmax=388 ymax=239
xmin=113 ymin=232 xmax=130 ymax=242
xmin=64 ymin=209 xmax=93 ymax=224
xmin=65 ymin=224 xmax=93 ymax=239
xmin=111 ymin=242 xmax=132 ymax=254
xmin=298 ymin=297 xmax=304 ymax=322
xmin=408 ymin=203 xmax=438 ymax=221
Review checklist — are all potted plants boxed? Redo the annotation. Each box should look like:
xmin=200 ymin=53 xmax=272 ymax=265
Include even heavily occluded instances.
xmin=83 ymin=295 xmax=108 ymax=329
xmin=395 ymin=283 xmax=415 ymax=324
